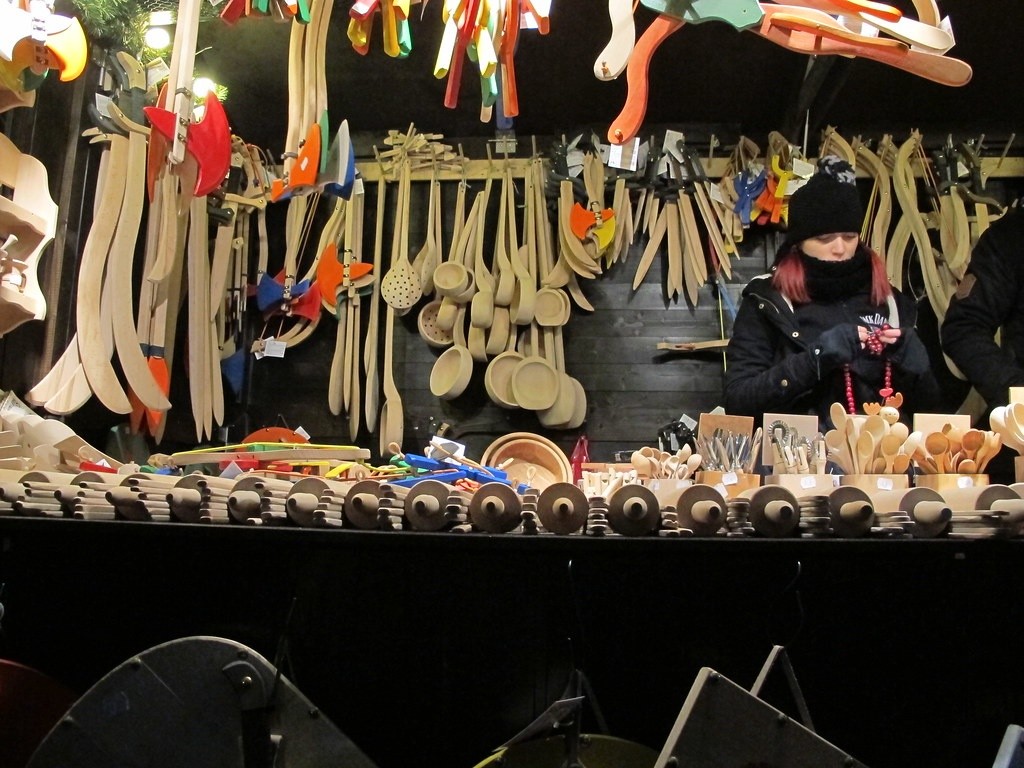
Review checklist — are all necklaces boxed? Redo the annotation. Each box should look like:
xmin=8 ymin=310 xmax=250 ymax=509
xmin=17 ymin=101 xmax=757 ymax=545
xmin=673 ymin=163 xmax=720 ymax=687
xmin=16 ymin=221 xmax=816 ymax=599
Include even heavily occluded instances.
xmin=846 ymin=323 xmax=898 ymax=416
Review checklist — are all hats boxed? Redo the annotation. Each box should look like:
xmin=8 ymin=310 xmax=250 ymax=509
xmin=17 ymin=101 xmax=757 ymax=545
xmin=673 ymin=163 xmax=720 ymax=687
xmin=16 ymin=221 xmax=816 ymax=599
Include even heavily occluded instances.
xmin=767 ymin=155 xmax=865 ymax=272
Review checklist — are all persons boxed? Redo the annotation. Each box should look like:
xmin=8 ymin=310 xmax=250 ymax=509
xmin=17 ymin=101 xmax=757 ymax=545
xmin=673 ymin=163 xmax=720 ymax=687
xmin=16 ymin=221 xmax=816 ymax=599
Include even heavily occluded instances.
xmin=940 ymin=205 xmax=1024 ymax=409
xmin=722 ymin=171 xmax=954 ymax=415
